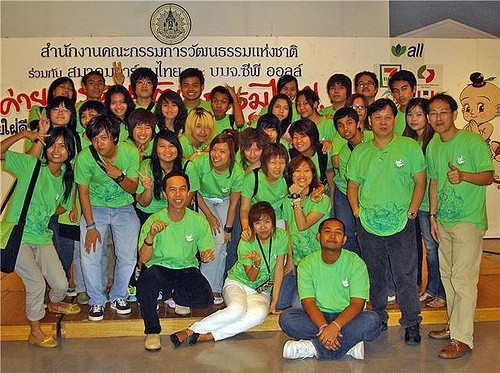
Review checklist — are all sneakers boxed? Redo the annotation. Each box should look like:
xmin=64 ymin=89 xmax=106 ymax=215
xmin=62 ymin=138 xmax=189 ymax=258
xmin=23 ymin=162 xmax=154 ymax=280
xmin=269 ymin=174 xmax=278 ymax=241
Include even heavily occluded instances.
xmin=345 ymin=340 xmax=365 ymax=360
xmin=88 ymin=305 xmax=105 ymax=321
xmin=109 ymin=298 xmax=132 ymax=315
xmin=282 ymin=339 xmax=319 ymax=361
xmin=126 ymin=296 xmax=137 ymax=302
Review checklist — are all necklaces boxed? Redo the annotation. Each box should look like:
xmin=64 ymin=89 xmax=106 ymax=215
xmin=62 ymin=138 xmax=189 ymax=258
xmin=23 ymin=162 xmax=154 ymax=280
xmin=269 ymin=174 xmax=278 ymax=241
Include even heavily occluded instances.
xmin=359 ymin=132 xmax=365 ymax=144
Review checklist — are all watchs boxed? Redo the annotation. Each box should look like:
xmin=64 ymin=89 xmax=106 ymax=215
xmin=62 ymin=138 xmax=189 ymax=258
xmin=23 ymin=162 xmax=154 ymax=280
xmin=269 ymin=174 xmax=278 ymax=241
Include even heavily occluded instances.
xmin=292 ymin=193 xmax=301 ymax=199
xmin=116 ymin=174 xmax=124 ymax=183
xmin=224 ymin=225 xmax=233 ymax=233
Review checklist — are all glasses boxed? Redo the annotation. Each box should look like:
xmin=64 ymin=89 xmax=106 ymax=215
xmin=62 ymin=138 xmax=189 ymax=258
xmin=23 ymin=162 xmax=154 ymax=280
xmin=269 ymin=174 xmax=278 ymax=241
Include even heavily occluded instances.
xmin=349 ymin=105 xmax=367 ymax=109
xmin=358 ymin=81 xmax=376 ymax=87
xmin=136 ymin=79 xmax=153 ymax=87
xmin=50 ymin=107 xmax=70 ymax=113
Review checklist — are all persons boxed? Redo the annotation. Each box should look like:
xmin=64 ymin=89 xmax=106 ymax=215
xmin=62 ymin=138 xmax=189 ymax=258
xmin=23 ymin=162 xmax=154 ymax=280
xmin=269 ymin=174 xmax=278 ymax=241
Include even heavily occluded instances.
xmin=0 ymin=61 xmax=494 ymax=359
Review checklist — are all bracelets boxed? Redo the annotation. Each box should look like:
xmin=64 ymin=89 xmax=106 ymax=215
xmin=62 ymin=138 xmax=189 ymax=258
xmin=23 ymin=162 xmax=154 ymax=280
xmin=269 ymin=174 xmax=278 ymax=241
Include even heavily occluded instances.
xmin=291 ymin=205 xmax=303 ymax=211
xmin=293 ymin=197 xmax=301 ymax=202
xmin=196 ymin=149 xmax=202 ymax=155
xmin=144 ymin=238 xmax=153 ymax=246
xmin=252 ymin=262 xmax=260 ymax=269
xmin=332 ymin=321 xmax=341 ymax=330
xmin=319 ymin=324 xmax=327 ymax=331
xmin=407 ymin=212 xmax=416 ymax=217
xmin=86 ymin=222 xmax=96 ymax=228
xmin=428 ymin=214 xmax=436 ymax=219
xmin=87 ymin=225 xmax=96 ymax=230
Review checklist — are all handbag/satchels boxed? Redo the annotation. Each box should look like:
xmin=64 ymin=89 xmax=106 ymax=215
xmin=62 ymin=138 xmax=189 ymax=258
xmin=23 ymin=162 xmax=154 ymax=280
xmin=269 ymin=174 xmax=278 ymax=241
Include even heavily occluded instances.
xmin=57 ymin=197 xmax=82 ymax=242
xmin=0 ymin=221 xmax=26 ymax=273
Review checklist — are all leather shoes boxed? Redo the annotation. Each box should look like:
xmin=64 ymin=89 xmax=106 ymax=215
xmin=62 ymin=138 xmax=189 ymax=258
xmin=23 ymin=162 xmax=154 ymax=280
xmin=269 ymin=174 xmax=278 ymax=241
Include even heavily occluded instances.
xmin=428 ymin=328 xmax=450 ymax=340
xmin=438 ymin=339 xmax=472 ymax=359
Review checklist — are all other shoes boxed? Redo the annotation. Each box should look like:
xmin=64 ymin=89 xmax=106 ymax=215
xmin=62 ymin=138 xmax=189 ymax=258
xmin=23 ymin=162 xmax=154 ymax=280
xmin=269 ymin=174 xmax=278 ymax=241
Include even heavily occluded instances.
xmin=170 ymin=330 xmax=200 ymax=348
xmin=44 ymin=301 xmax=81 ymax=314
xmin=404 ymin=325 xmax=421 ymax=345
xmin=212 ymin=292 xmax=223 ymax=305
xmin=157 ymin=291 xmax=164 ymax=301
xmin=127 ymin=285 xmax=137 ymax=296
xmin=164 ymin=298 xmax=176 ymax=309
xmin=175 ymin=303 xmax=191 ymax=315
xmin=28 ymin=332 xmax=58 ymax=348
xmin=66 ymin=288 xmax=78 ymax=296
xmin=77 ymin=292 xmax=89 ymax=304
xmin=381 ymin=321 xmax=388 ymax=331
xmin=144 ymin=334 xmax=162 ymax=350
xmin=387 ymin=295 xmax=397 ymax=305
xmin=419 ymin=292 xmax=446 ymax=308
